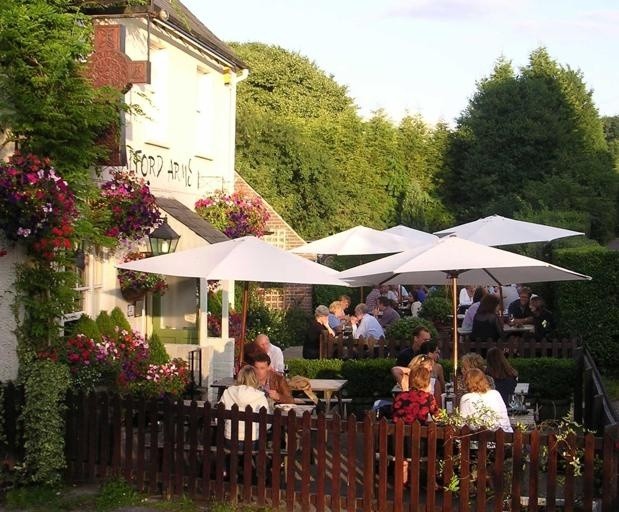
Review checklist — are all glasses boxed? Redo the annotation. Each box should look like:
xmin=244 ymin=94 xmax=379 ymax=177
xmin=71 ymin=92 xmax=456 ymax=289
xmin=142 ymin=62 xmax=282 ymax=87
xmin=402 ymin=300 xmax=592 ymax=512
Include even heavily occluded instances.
xmin=414 ymin=355 xmax=430 ymax=361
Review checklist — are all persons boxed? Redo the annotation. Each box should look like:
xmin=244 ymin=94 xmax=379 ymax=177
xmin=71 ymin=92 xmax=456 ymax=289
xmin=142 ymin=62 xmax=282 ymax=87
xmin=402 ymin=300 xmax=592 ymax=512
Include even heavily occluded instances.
xmin=393 ymin=327 xmax=430 ymax=366
xmin=390 ymin=368 xmax=442 ymax=458
xmin=390 ymin=354 xmax=443 ymax=413
xmin=242 ymin=344 xmax=268 ymax=366
xmin=485 ymin=346 xmax=518 ymax=408
xmin=529 ymin=296 xmax=563 ymax=346
xmin=460 ymin=285 xmax=489 ymax=351
xmin=402 ymin=291 xmax=423 ymax=318
xmin=419 ymin=341 xmax=447 ymax=388
xmin=335 ymin=294 xmax=350 ymax=310
xmin=214 ymin=365 xmax=271 ymax=486
xmin=326 ymin=300 xmax=346 ymax=329
xmin=302 ymin=305 xmax=339 ymax=359
xmin=250 ymin=354 xmax=294 ymax=406
xmin=458 ymin=367 xmax=514 ymax=462
xmin=253 ymin=334 xmax=285 ymax=374
xmin=460 ymin=285 xmax=478 ymax=306
xmin=365 ymin=284 xmax=380 ymax=311
xmin=505 ymin=285 xmax=538 ymax=330
xmin=467 ymin=293 xmax=505 ymax=345
xmin=349 ymin=303 xmax=388 ymax=360
xmin=493 ymin=283 xmax=519 ymax=310
xmin=453 ymin=353 xmax=495 ymax=410
xmin=371 ymin=296 xmax=402 ymax=329
xmin=378 ymin=282 xmax=399 ymax=304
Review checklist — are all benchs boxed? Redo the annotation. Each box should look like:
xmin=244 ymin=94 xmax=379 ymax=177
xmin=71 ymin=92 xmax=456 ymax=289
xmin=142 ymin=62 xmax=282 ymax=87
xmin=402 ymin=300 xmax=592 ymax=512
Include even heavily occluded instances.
xmin=292 ymin=397 xmax=353 ymax=437
xmin=373 ymin=449 xmax=447 ymax=492
xmin=294 ymin=413 xmax=335 ymax=455
xmin=144 ymin=439 xmax=297 ymax=489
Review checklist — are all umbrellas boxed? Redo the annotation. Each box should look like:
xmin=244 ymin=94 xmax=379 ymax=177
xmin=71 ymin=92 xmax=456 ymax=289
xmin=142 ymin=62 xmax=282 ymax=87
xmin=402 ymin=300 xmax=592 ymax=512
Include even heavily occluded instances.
xmin=441 ymin=215 xmax=586 ymax=246
xmin=114 ymin=236 xmax=351 ymax=373
xmin=334 ymin=236 xmax=592 ymax=416
xmin=289 ymin=225 xmax=420 ymax=256
xmin=433 ymin=220 xmax=486 ymax=237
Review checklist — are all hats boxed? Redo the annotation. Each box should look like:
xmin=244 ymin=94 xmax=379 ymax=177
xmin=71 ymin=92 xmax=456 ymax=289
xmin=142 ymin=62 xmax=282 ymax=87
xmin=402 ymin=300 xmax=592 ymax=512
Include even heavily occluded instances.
xmin=419 ymin=341 xmax=437 ymax=354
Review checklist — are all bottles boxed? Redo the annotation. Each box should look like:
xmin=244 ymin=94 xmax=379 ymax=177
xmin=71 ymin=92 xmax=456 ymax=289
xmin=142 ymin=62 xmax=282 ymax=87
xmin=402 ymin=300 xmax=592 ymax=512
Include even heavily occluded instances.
xmin=284 ymin=364 xmax=289 ymax=378
xmin=444 ymin=391 xmax=452 ymax=415
